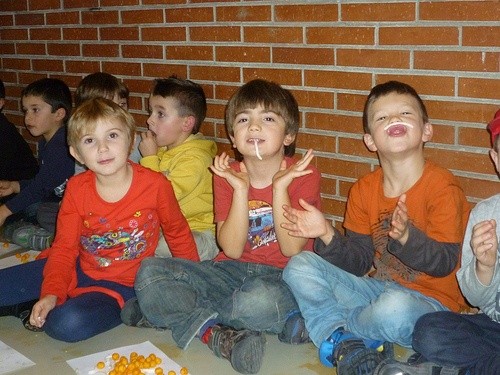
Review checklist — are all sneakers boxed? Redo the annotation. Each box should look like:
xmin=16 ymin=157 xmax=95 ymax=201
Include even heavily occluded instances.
xmin=12 ymin=226 xmax=55 ymax=251
xmin=318 ymin=329 xmax=394 ymax=375
xmin=278 ymin=310 xmax=311 ymax=344
xmin=209 ymin=324 xmax=266 ymax=375
xmin=120 ymin=297 xmax=172 ymax=329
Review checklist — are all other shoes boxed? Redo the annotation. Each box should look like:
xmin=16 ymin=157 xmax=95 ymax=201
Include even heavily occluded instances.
xmin=374 ymin=357 xmax=462 ymax=375
xmin=13 ymin=302 xmax=45 ymax=333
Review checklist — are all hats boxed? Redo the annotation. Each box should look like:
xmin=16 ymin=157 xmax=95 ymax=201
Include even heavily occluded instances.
xmin=487 ymin=109 xmax=500 ymax=146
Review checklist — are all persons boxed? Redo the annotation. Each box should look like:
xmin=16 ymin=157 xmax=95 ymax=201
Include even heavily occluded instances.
xmin=0 ymin=72 xmax=219 ymax=260
xmin=375 ymin=110 xmax=500 ymax=375
xmin=282 ymin=81 xmax=469 ymax=375
xmin=120 ymin=81 xmax=319 ymax=375
xmin=0 ymin=100 xmax=200 ymax=340
